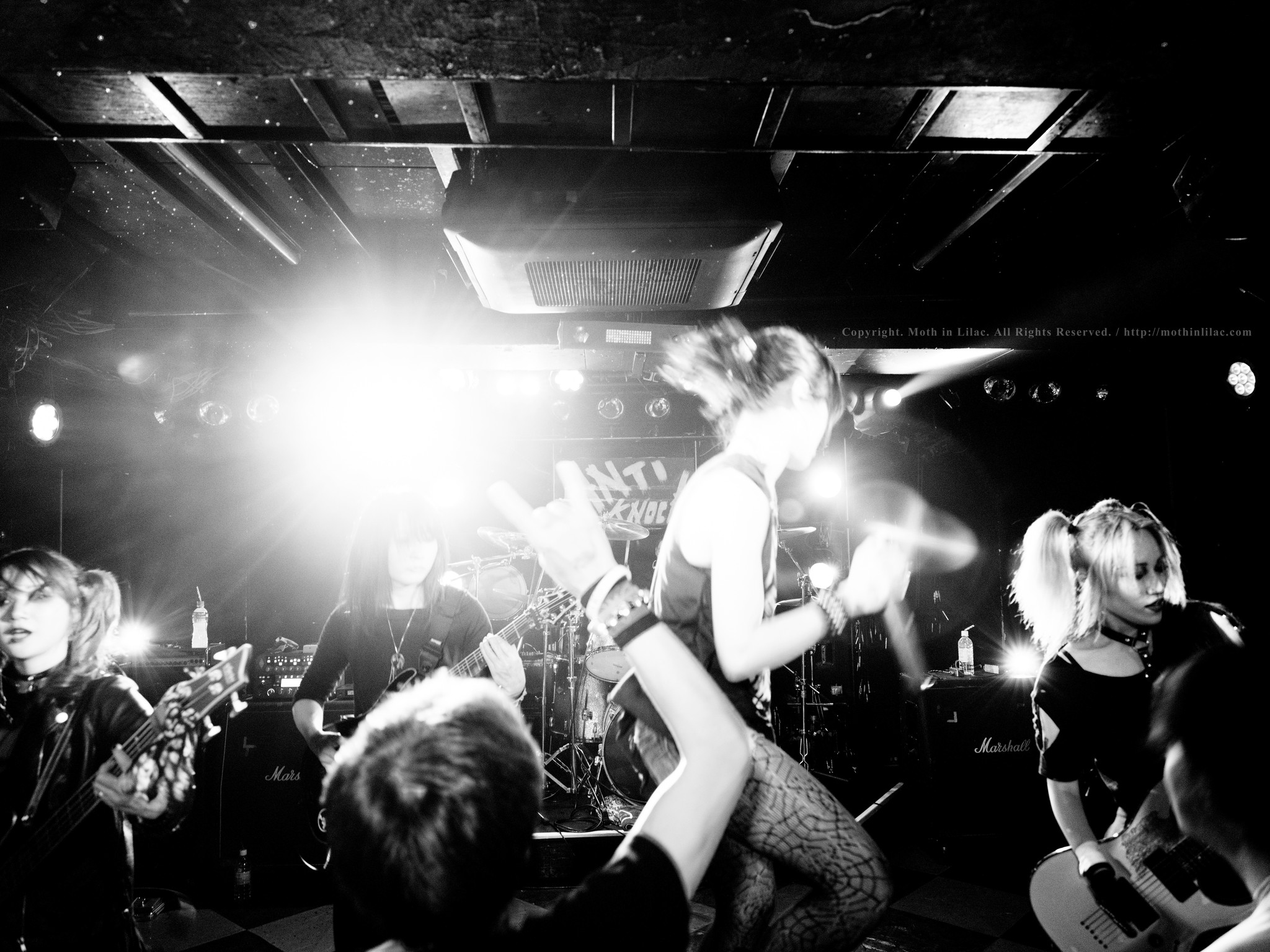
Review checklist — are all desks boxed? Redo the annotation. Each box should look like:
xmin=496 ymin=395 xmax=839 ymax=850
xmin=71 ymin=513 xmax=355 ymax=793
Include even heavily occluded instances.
xmin=203 ymin=692 xmax=356 ymax=881
xmin=108 ymin=642 xmax=226 ymax=708
xmin=919 ymin=668 xmax=1070 ymax=870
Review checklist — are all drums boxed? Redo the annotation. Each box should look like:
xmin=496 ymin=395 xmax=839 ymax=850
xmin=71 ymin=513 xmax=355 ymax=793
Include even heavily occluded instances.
xmin=551 ymin=643 xmax=657 ymax=805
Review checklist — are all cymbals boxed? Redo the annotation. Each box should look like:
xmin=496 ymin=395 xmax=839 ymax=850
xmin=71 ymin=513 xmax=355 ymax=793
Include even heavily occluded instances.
xmin=598 ymin=517 xmax=651 ymax=543
xmin=775 ymin=525 xmax=817 ymax=543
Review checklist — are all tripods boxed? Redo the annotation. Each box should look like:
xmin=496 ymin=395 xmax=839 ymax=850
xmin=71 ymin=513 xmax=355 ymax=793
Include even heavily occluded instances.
xmin=543 ymin=611 xmax=612 ymax=794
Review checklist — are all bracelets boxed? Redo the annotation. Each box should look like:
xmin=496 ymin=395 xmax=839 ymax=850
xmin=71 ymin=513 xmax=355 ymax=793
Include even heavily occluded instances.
xmin=586 ymin=567 xmax=646 ymax=629
xmin=812 ymin=589 xmax=849 ymax=636
xmin=515 ymin=688 xmax=526 ymax=704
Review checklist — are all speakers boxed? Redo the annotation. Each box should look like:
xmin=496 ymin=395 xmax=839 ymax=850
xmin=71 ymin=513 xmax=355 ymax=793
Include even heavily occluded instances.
xmin=889 ymin=659 xmax=1067 ymax=882
xmin=216 ymin=699 xmax=358 ymax=891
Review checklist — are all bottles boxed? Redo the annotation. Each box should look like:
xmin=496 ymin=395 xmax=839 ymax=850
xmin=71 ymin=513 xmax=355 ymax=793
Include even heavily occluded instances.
xmin=191 ymin=601 xmax=209 ymax=648
xmin=958 ymin=631 xmax=974 ymax=675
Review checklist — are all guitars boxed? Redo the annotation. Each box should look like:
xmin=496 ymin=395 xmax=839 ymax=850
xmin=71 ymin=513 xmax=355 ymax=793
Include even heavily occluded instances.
xmin=0 ymin=641 xmax=253 ymax=888
xmin=1026 ymin=771 xmax=1261 ymax=952
xmin=322 ymin=587 xmax=578 ymax=756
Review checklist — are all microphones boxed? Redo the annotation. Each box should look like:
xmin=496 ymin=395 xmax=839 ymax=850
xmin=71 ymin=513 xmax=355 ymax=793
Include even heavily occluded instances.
xmin=883 ymin=595 xmax=928 ymax=690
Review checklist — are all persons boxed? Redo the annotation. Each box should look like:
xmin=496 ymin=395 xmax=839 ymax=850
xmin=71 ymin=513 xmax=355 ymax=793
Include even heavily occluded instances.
xmin=1010 ymin=492 xmax=1250 ymax=952
xmin=2 ymin=544 xmax=159 ymax=952
xmin=291 ymin=489 xmax=526 ymax=778
xmin=1147 ymin=644 xmax=1270 ymax=952
xmin=319 ymin=460 xmax=755 ymax=951
xmin=603 ymin=316 xmax=897 ymax=952
